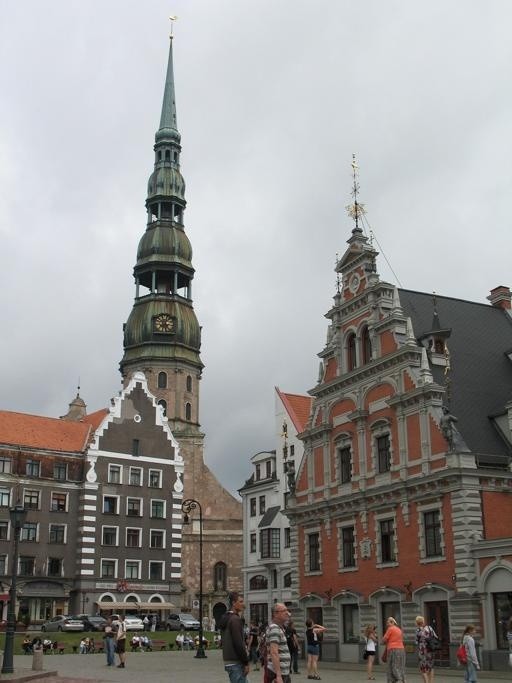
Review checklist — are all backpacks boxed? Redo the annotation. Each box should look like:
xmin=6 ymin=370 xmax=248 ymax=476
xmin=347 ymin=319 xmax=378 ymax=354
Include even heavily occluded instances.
xmin=456 ymin=643 xmax=468 ymax=665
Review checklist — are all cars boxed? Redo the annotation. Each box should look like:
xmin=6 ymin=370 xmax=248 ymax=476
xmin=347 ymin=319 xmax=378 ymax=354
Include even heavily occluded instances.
xmin=111 ymin=613 xmax=145 ymax=631
xmin=75 ymin=613 xmax=111 ymax=631
xmin=39 ymin=613 xmax=85 ymax=632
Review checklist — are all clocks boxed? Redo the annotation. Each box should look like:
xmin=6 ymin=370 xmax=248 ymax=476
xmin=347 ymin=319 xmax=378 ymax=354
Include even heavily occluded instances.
xmin=154 ymin=313 xmax=174 ymax=332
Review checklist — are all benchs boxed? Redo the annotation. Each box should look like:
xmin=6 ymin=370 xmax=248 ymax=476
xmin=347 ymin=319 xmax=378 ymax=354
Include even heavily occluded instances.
xmin=71 ymin=641 xmax=105 ymax=652
xmin=175 ymin=638 xmax=210 ymax=650
xmin=215 ymin=639 xmax=222 ymax=649
xmin=129 ymin=639 xmax=168 ymax=652
xmin=21 ymin=642 xmax=65 ymax=654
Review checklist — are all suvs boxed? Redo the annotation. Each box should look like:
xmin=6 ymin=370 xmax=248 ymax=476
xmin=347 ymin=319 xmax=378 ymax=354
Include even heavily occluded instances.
xmin=164 ymin=611 xmax=200 ymax=631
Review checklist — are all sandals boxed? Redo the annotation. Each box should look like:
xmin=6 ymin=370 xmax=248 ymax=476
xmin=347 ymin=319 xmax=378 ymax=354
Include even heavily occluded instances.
xmin=368 ymin=675 xmax=376 ymax=680
xmin=307 ymin=674 xmax=321 ymax=680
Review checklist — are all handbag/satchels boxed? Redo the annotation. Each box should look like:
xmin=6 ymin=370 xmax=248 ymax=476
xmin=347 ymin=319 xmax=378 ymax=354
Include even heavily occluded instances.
xmin=362 ymin=651 xmax=369 ymax=659
xmin=426 ymin=627 xmax=443 ymax=651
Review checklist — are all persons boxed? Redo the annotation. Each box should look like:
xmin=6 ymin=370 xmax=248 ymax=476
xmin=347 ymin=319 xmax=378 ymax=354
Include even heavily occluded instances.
xmin=379 ymin=617 xmax=407 ymax=683
xmin=415 ymin=616 xmax=439 ymax=683
xmin=506 ymin=617 xmax=512 ymax=665
xmin=305 ymin=618 xmax=328 ymax=680
xmin=461 ymin=624 xmax=480 ymax=683
xmin=365 ymin=624 xmax=379 ymax=680
xmin=284 ymin=460 xmax=296 ymax=497
xmin=21 ymin=592 xmax=303 ymax=683
xmin=438 ymin=406 xmax=460 ymax=452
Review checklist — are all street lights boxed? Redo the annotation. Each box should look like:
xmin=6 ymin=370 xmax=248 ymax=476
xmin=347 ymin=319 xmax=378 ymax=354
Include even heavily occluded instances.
xmin=0 ymin=496 xmax=31 ymax=673
xmin=180 ymin=497 xmax=210 ymax=659
